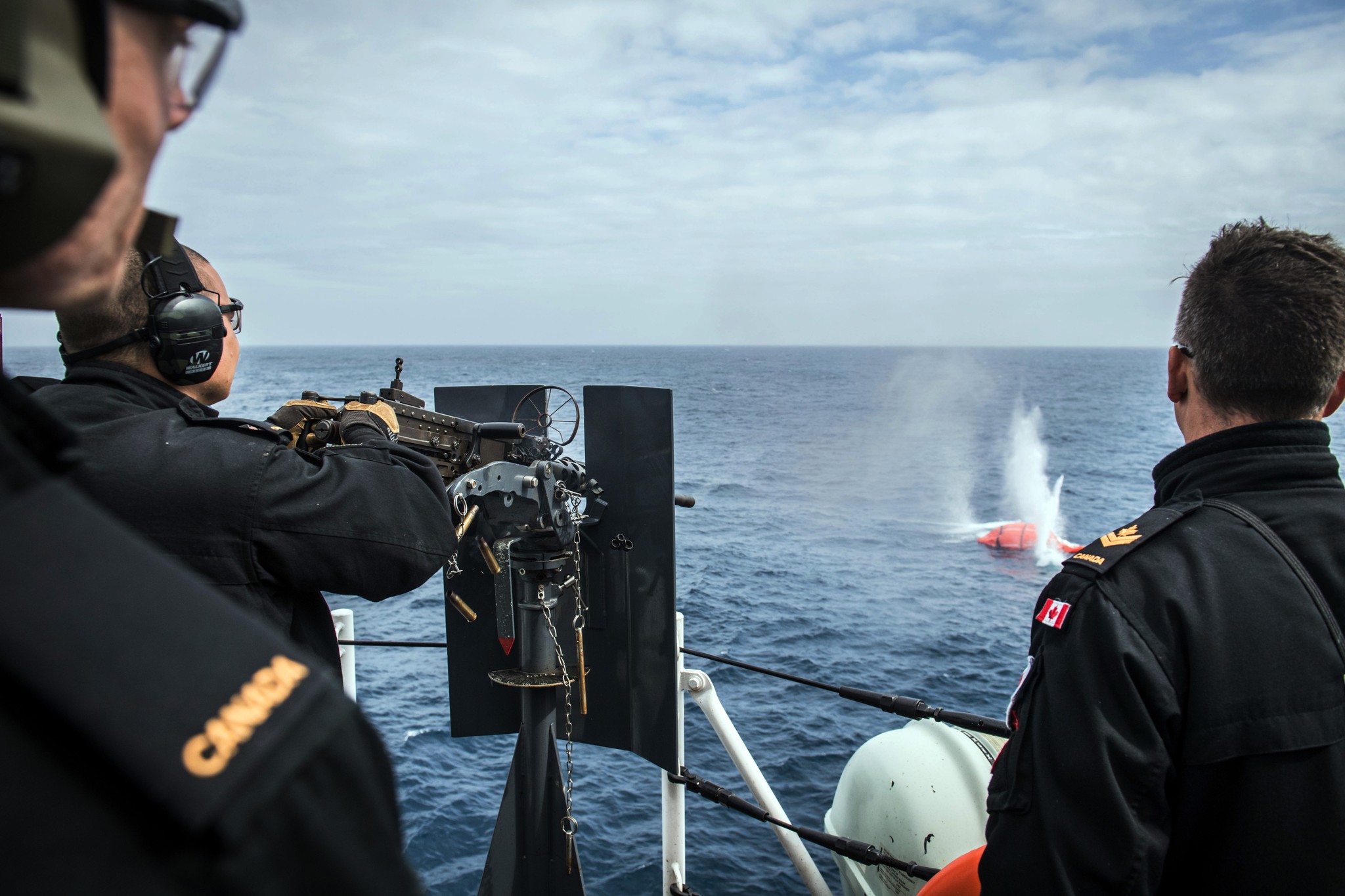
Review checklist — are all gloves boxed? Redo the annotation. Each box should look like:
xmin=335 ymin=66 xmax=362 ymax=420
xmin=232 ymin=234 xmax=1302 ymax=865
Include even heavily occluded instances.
xmin=267 ymin=399 xmax=337 ymax=452
xmin=339 ymin=400 xmax=400 ymax=445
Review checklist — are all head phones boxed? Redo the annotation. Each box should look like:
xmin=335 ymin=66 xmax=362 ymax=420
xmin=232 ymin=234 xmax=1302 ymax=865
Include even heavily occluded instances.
xmin=141 ymin=236 xmax=227 ymax=386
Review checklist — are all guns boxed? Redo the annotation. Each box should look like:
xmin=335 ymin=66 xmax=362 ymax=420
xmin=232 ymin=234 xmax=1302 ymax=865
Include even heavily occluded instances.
xmin=294 ymin=383 xmax=696 ymax=555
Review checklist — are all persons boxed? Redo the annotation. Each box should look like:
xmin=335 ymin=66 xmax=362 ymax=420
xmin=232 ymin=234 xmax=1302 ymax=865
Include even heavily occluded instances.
xmin=977 ymin=220 xmax=1345 ymax=896
xmin=0 ymin=0 xmax=459 ymax=896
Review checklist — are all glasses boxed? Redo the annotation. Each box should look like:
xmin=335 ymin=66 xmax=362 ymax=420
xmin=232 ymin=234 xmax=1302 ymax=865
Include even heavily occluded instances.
xmin=121 ymin=0 xmax=243 ymax=109
xmin=219 ymin=298 xmax=243 ymax=334
